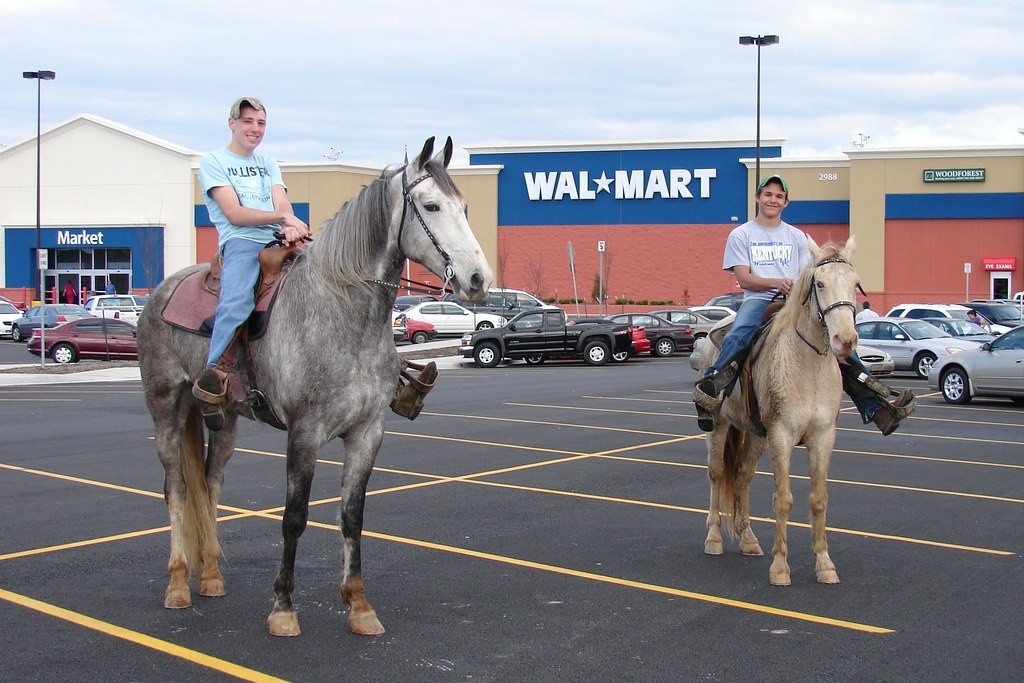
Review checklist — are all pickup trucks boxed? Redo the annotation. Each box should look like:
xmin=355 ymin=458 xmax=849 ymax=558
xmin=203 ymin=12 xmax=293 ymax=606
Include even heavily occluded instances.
xmin=457 ymin=307 xmax=633 ymax=369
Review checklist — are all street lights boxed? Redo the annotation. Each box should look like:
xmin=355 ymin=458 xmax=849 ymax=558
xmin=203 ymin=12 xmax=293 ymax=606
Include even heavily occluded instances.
xmin=22 ymin=69 xmax=58 ymax=369
xmin=737 ymin=33 xmax=782 ymax=218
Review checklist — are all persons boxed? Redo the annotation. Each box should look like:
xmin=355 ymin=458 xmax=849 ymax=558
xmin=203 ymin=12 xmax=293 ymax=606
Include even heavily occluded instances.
xmin=198 ymin=96 xmax=439 ymax=431
xmin=694 ymin=175 xmax=914 ymax=436
xmin=63 ymin=280 xmax=76 ymax=304
xmin=967 ymin=310 xmax=984 ymax=327
xmin=105 ymin=280 xmax=116 ymax=295
xmin=856 ymin=301 xmax=880 ymax=322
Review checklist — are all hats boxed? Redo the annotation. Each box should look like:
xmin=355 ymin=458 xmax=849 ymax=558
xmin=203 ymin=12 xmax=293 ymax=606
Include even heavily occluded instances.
xmin=230 ymin=97 xmax=266 ymax=118
xmin=758 ymin=175 xmax=789 ymax=196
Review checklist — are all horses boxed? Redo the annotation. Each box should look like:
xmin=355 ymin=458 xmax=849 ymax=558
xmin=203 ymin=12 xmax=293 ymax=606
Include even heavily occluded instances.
xmin=692 ymin=232 xmax=860 ymax=588
xmin=138 ymin=135 xmax=492 ymax=637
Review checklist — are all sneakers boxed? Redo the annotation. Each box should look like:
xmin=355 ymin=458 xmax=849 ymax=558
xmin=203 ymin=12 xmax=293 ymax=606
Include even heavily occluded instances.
xmin=390 ymin=361 xmax=437 ymax=420
xmin=872 ymin=388 xmax=912 ymax=436
xmin=695 ymin=381 xmax=716 ymax=432
xmin=199 ymin=369 xmax=226 ymax=432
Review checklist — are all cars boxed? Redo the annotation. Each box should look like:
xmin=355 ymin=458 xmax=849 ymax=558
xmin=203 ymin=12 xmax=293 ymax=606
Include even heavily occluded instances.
xmin=856 ymin=317 xmax=984 ymax=380
xmin=672 ymin=305 xmax=738 ymax=322
xmin=689 ymin=335 xmax=895 ymax=376
xmin=0 ymin=295 xmax=29 ymax=336
xmin=83 ymin=293 xmax=150 ymax=327
xmin=391 ymin=300 xmax=508 ymax=344
xmin=633 ymin=309 xmax=719 ymax=353
xmin=11 ymin=302 xmax=98 ymax=343
xmin=26 ymin=316 xmax=138 ymax=363
xmin=885 ymin=291 xmax=1024 ymax=343
xmin=543 ymin=316 xmax=651 ymax=363
xmin=927 ymin=324 xmax=1024 ymax=406
xmin=602 ymin=312 xmax=696 ymax=358
xmin=393 ymin=287 xmax=568 ymax=326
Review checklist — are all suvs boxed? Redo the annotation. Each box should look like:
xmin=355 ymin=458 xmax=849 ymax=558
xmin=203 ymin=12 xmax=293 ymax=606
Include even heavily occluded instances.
xmin=704 ymin=292 xmax=744 ymax=312
